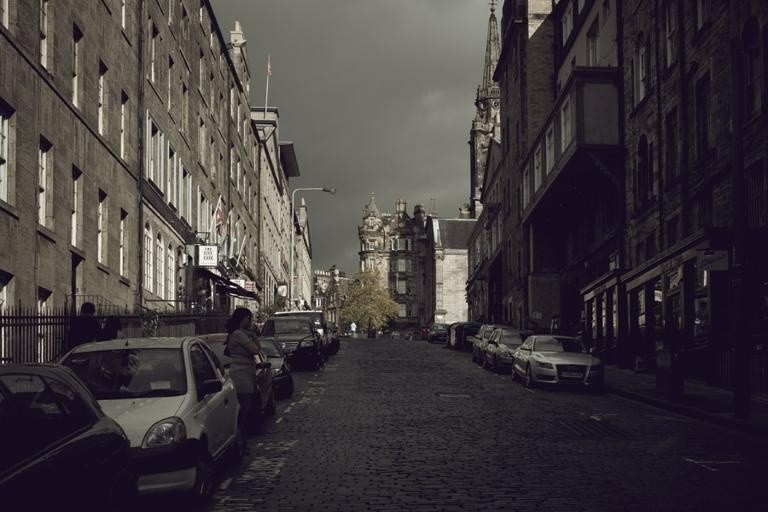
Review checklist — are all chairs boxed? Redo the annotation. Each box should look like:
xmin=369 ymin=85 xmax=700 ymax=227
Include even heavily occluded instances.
xmin=150 ymin=362 xmax=182 ymax=387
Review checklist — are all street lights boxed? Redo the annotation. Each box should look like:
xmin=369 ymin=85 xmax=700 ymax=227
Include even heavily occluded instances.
xmin=286 ymin=186 xmax=335 ymax=310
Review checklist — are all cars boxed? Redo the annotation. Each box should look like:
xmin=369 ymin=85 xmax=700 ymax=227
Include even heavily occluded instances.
xmin=427 ymin=323 xmax=450 ymax=343
xmin=262 ymin=316 xmax=320 ymax=369
xmin=508 ymin=333 xmax=603 ymax=388
xmin=30 ymin=335 xmax=244 ymax=501
xmin=473 ymin=325 xmax=534 ymax=372
xmin=0 ymin=361 xmax=140 ymax=512
xmin=327 ymin=321 xmax=339 ymax=355
xmin=198 ymin=331 xmax=293 ymax=421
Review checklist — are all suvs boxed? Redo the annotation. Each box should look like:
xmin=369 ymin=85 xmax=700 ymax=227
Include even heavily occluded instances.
xmin=276 ymin=308 xmax=328 ymax=360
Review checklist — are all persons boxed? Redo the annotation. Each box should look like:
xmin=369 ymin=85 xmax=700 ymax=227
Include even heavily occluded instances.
xmin=223 ymin=304 xmax=268 ymax=437
xmin=101 ymin=315 xmax=123 ymax=340
xmin=65 ymin=299 xmax=102 ymax=352
xmin=350 ymin=321 xmax=357 ymax=337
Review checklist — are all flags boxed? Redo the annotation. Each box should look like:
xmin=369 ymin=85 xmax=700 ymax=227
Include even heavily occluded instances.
xmin=266 ymin=56 xmax=272 ymax=75
